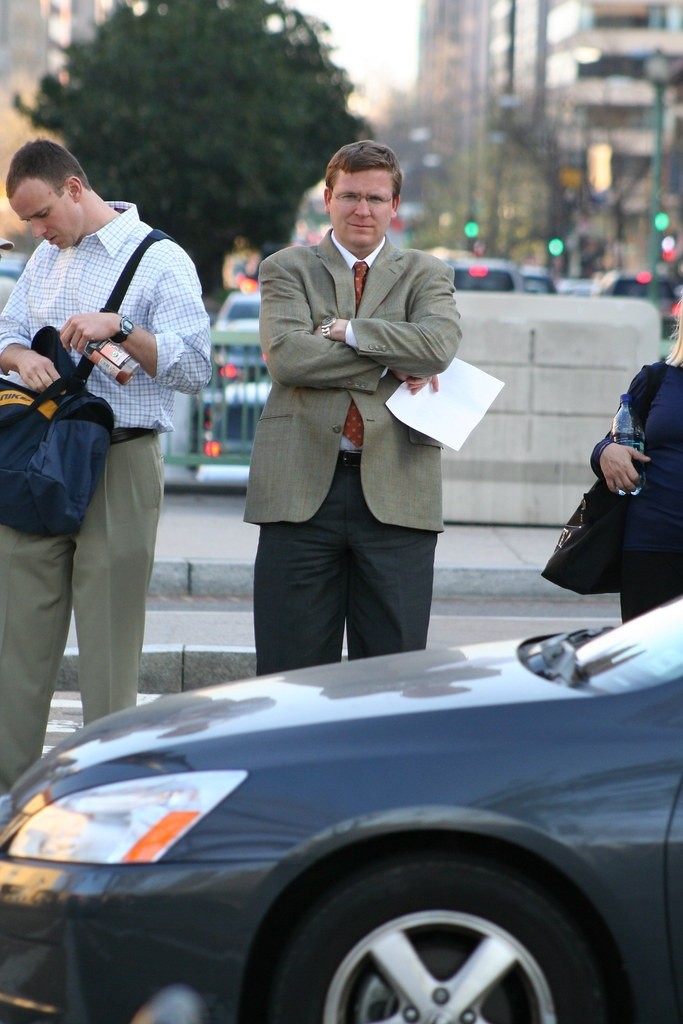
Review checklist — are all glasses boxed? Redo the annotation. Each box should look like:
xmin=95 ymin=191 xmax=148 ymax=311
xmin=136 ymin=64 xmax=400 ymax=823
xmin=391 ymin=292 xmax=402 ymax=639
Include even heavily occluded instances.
xmin=329 ymin=188 xmax=395 ymax=207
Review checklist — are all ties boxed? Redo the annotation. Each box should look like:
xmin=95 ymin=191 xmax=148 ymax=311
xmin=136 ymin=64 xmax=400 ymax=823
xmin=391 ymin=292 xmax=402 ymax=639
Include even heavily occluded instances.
xmin=341 ymin=261 xmax=369 ymax=448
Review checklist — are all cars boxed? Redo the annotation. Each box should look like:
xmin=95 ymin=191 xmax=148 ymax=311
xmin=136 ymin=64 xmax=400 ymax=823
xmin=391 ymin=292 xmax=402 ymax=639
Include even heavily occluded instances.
xmin=432 ymin=249 xmax=681 ymax=339
xmin=0 ymin=588 xmax=681 ymax=1024
xmin=194 ymin=287 xmax=274 ymax=462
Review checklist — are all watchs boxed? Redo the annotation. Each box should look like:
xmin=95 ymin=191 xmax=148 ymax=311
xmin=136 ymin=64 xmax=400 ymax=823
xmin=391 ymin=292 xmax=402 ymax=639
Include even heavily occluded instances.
xmin=109 ymin=315 xmax=136 ymax=345
xmin=320 ymin=314 xmax=338 ymax=341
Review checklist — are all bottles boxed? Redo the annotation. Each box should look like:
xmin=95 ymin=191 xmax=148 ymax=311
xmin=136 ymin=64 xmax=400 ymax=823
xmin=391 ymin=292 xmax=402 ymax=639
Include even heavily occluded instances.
xmin=612 ymin=394 xmax=647 ymax=495
xmin=82 ymin=338 xmax=141 ymax=386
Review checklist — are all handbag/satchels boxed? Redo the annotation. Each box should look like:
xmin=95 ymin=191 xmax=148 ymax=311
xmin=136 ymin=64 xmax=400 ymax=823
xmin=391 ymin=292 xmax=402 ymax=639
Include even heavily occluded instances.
xmin=0 ymin=326 xmax=114 ymax=534
xmin=542 ymin=362 xmax=668 ymax=596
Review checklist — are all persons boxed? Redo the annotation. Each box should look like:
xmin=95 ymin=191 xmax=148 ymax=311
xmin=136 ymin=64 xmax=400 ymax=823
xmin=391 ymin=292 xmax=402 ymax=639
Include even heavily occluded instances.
xmin=0 ymin=139 xmax=213 ymax=795
xmin=591 ymin=292 xmax=683 ymax=624
xmin=241 ymin=140 xmax=463 ymax=679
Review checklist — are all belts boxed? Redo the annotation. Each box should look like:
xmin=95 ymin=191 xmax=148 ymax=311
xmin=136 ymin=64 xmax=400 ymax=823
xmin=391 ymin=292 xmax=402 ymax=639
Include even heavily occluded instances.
xmin=336 ymin=448 xmax=363 ymax=467
xmin=109 ymin=427 xmax=157 ymax=444
